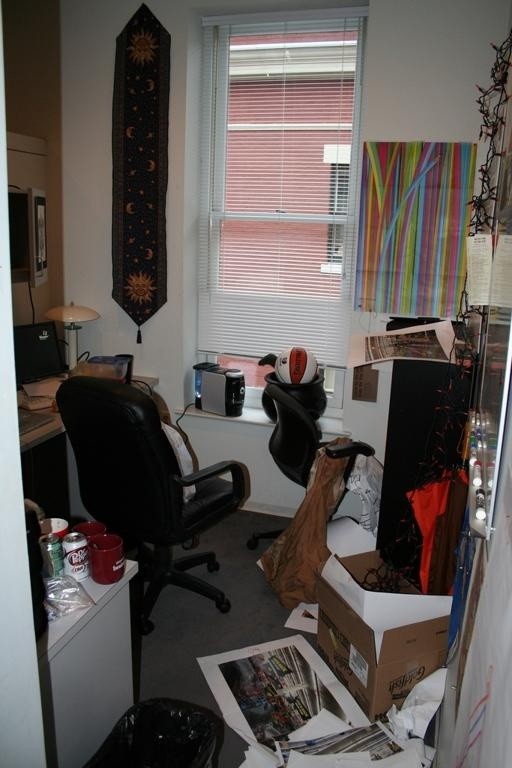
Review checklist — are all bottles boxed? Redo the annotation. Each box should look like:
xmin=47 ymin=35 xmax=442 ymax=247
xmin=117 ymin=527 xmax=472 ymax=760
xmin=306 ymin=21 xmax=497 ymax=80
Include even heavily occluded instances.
xmin=61 ymin=532 xmax=90 ymax=583
xmin=39 ymin=534 xmax=64 ymax=578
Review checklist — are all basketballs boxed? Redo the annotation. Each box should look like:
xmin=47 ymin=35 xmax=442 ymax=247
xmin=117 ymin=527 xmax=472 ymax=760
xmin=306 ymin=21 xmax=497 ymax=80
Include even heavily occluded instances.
xmin=275 ymin=347 xmax=318 ymax=385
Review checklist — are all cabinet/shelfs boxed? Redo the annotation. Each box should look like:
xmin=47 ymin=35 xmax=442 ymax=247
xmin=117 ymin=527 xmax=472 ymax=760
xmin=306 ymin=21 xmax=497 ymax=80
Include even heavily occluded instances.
xmin=342 ymin=316 xmax=479 ymax=592
xmin=6 ymin=130 xmax=66 ymax=327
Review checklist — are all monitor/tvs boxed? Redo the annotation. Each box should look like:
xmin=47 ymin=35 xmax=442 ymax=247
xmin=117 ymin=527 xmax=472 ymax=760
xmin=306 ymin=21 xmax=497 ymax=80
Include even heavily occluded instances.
xmin=14 ymin=321 xmax=70 ymax=393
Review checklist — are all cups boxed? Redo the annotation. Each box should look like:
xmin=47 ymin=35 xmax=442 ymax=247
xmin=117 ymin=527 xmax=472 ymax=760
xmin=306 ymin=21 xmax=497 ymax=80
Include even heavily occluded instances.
xmin=88 ymin=534 xmax=124 ymax=584
xmin=72 ymin=521 xmax=106 ymax=538
xmin=40 ymin=518 xmax=68 ymax=537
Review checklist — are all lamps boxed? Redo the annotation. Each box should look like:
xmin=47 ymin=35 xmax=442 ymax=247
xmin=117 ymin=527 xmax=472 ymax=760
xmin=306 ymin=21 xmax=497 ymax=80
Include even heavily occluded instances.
xmin=44 ymin=300 xmax=101 ymax=372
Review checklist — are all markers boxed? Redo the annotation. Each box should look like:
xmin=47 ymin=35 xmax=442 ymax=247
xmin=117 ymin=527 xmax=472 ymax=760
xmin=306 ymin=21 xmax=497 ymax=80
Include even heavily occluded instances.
xmin=486 ymin=488 xmax=493 ymax=515
xmin=473 ymin=459 xmax=483 ymax=486
xmin=468 ymin=449 xmax=478 ymax=466
xmin=472 ymin=410 xmax=475 ymax=423
xmin=486 ymin=462 xmax=495 ymax=486
xmin=477 ymin=429 xmax=483 ymax=448
xmin=475 ymin=489 xmax=485 ymax=520
xmin=470 ymin=430 xmax=475 ymax=450
xmin=483 ymin=430 xmax=486 ymax=449
xmin=476 ymin=410 xmax=482 ymax=426
xmin=482 ymin=408 xmax=490 ymax=426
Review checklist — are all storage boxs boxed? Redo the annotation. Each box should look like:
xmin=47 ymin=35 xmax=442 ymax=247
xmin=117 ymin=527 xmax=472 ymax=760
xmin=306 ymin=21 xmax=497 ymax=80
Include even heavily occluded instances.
xmin=321 ymin=549 xmax=454 ymax=664
xmin=311 ymin=569 xmax=450 ymax=724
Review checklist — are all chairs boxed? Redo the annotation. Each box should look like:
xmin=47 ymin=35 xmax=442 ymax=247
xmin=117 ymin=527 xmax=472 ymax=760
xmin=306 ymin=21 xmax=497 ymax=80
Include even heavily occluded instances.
xmin=247 ymin=384 xmax=375 ymax=550
xmin=56 ymin=375 xmax=245 ymax=634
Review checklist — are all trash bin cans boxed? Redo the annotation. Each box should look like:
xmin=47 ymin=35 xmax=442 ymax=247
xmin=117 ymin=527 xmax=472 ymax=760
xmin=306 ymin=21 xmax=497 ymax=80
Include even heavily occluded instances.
xmin=83 ymin=696 xmax=226 ymax=767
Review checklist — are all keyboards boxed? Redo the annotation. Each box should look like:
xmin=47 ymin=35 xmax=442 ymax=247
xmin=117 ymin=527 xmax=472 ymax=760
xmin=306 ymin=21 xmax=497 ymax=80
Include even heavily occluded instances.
xmin=21 ymin=394 xmax=60 ymax=412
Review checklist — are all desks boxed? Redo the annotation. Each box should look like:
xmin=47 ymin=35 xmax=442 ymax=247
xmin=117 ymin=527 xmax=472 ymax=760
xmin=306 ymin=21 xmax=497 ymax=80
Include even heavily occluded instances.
xmin=38 ymin=559 xmax=138 ymax=767
xmin=18 ymin=373 xmax=159 ymax=453
